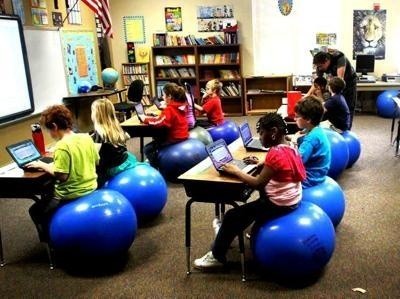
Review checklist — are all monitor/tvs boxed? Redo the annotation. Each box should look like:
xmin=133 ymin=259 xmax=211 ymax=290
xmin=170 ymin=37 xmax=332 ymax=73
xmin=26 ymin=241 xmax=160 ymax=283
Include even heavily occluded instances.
xmin=356 ymin=55 xmax=374 ymax=75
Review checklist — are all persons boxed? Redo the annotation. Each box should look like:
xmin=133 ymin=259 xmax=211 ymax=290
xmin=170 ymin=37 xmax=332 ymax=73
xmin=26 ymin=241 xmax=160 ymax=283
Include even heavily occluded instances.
xmin=310 ymin=75 xmax=351 ymax=134
xmin=288 ymin=94 xmax=333 ymax=190
xmin=171 ymin=76 xmax=197 ymax=130
xmin=29 ymin=105 xmax=101 ymax=259
xmin=139 ymin=82 xmax=189 ymax=170
xmin=310 ymin=76 xmax=331 ymax=101
xmin=87 ymin=98 xmax=137 ymax=188
xmin=192 ymin=77 xmax=225 ymax=127
xmin=302 ymin=49 xmax=358 ymax=132
xmin=190 ymin=112 xmax=303 ymax=270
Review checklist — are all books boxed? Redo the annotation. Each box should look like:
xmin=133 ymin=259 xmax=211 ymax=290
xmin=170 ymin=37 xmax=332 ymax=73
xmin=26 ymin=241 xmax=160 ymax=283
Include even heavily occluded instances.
xmin=151 ymin=30 xmax=242 ymax=99
xmin=122 ymin=63 xmax=152 ymax=114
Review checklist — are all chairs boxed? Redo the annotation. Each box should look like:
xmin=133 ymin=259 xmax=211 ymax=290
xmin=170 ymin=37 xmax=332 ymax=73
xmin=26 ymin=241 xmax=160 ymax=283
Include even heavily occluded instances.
xmin=113 ymin=80 xmax=144 ymax=113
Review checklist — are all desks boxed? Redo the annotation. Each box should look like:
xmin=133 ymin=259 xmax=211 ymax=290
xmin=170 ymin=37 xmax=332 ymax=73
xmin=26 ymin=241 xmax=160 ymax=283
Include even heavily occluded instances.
xmin=176 ymin=135 xmax=271 ymax=284
xmin=293 ymin=77 xmax=400 ymax=112
xmin=120 ymin=107 xmax=165 ymax=163
xmin=61 ymin=86 xmax=127 ymax=119
xmin=0 ymin=146 xmax=63 ymax=272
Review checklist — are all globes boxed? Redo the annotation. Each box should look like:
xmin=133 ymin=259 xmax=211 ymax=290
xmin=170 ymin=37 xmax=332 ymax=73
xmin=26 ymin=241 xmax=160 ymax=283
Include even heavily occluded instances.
xmin=101 ymin=68 xmax=119 ymax=90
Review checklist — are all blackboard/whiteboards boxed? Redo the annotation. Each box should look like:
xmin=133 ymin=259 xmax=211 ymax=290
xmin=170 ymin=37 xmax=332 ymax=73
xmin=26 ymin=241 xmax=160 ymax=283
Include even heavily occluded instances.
xmin=0 ymin=25 xmax=70 ymax=130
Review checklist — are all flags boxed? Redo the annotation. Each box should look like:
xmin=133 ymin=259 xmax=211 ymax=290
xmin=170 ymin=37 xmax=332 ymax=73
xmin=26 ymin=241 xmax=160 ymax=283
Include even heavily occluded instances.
xmin=80 ymin=0 xmax=114 ymax=40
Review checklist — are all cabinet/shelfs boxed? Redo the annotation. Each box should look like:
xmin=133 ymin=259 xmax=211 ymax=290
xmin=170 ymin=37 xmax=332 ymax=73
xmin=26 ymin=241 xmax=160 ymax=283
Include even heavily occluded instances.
xmin=122 ymin=63 xmax=153 ymax=109
xmin=243 ymin=75 xmax=294 ymax=116
xmin=152 ymin=44 xmax=245 ymax=117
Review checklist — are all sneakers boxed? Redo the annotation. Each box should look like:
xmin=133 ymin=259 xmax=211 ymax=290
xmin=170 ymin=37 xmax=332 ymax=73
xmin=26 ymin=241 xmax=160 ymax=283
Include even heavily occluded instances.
xmin=194 ymin=251 xmax=224 ymax=269
xmin=213 ymin=218 xmax=225 ymax=236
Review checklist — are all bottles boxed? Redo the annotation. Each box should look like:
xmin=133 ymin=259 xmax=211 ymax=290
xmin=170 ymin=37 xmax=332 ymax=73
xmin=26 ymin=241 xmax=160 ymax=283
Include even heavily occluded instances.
xmin=30 ymin=123 xmax=45 ymax=155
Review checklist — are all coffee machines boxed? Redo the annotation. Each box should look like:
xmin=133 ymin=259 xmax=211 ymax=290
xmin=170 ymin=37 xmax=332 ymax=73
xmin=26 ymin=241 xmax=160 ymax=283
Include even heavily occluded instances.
xmin=222 ymin=18 xmax=238 ymax=46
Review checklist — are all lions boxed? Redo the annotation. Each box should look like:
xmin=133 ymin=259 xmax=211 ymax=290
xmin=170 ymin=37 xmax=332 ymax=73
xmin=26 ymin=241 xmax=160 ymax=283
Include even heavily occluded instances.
xmin=353 ymin=10 xmax=386 ymax=57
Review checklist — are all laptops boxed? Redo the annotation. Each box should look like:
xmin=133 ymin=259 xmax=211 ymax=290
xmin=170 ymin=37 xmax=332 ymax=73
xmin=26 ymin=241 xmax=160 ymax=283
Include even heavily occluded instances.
xmin=152 ymin=97 xmax=162 ymax=109
xmin=206 ymin=139 xmax=258 ymax=176
xmin=134 ymin=103 xmax=157 ymax=123
xmin=239 ymin=120 xmax=270 ymax=151
xmin=5 ymin=138 xmax=54 ymax=172
